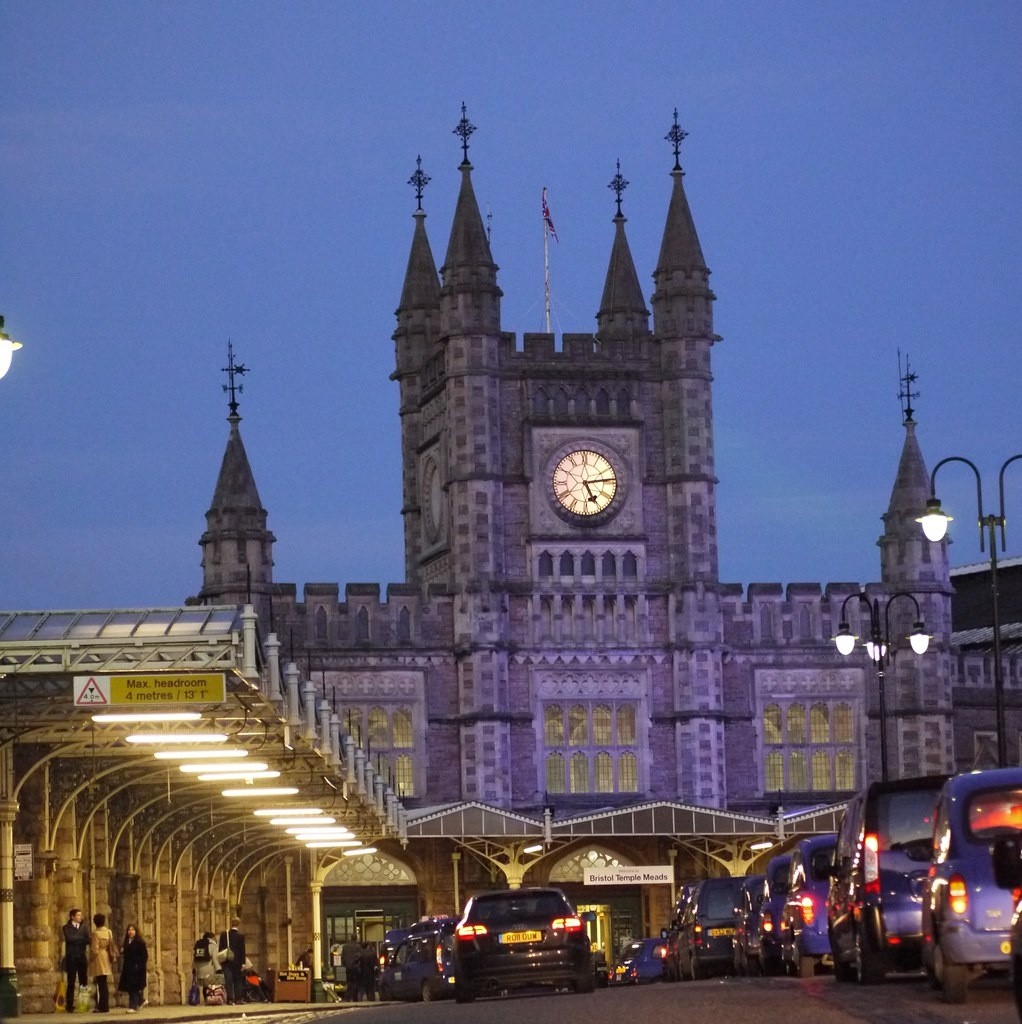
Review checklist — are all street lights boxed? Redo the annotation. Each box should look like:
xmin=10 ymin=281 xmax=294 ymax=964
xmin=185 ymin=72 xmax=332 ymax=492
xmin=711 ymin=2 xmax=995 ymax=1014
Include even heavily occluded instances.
xmin=915 ymin=455 xmax=1022 ymax=766
xmin=831 ymin=592 xmax=933 ymax=781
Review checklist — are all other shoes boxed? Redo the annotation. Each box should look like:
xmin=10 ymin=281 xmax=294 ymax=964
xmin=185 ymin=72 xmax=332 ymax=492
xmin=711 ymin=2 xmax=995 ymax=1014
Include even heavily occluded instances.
xmin=91 ymin=1008 xmax=110 ymax=1014
xmin=126 ymin=1008 xmax=136 ymax=1013
xmin=229 ymin=999 xmax=234 ymax=1004
xmin=236 ymin=1000 xmax=246 ymax=1004
xmin=139 ymin=1000 xmax=149 ymax=1010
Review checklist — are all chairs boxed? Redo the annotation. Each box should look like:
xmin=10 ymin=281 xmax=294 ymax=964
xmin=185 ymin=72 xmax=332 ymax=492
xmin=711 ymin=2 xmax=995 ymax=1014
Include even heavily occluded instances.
xmin=531 ymin=899 xmax=552 ymax=917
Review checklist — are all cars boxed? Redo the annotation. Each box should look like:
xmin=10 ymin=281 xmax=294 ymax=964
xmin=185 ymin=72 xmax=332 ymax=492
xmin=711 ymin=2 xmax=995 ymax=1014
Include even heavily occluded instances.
xmin=375 ymin=932 xmax=452 ymax=1002
xmin=452 ymin=889 xmax=598 ymax=1004
xmin=784 ymin=834 xmax=847 ymax=981
xmin=734 ymin=854 xmax=799 ymax=980
xmin=905 ymin=768 xmax=1022 ymax=1003
xmin=607 ymin=939 xmax=671 ymax=986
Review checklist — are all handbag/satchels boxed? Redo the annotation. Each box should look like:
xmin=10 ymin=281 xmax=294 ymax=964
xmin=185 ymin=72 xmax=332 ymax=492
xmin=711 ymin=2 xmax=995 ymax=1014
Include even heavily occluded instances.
xmin=55 ymin=982 xmax=66 ymax=1010
xmin=62 ymin=959 xmax=67 ymax=972
xmin=106 ymin=930 xmax=120 ymax=963
xmin=207 ymin=983 xmax=226 ymax=1005
xmin=217 ymin=948 xmax=234 ymax=964
xmin=189 ymin=971 xmax=200 ymax=1005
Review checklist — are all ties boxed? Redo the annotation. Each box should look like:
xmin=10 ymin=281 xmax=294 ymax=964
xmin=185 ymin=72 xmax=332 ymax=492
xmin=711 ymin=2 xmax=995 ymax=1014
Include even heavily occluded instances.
xmin=75 ymin=924 xmax=77 ymax=928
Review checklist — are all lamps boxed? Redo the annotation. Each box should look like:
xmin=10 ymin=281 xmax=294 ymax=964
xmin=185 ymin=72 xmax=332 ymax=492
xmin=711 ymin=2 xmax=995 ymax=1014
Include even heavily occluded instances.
xmin=92 ymin=709 xmax=379 ymax=856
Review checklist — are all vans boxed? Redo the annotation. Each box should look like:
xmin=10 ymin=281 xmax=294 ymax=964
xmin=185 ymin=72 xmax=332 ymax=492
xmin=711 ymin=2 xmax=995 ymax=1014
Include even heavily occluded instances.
xmin=813 ymin=774 xmax=957 ymax=986
xmin=662 ymin=878 xmax=749 ymax=982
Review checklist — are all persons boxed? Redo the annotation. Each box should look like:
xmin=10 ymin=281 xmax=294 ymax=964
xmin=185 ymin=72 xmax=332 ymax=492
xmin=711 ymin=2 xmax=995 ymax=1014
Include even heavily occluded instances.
xmin=361 ymin=941 xmax=382 ymax=1002
xmin=89 ymin=913 xmax=114 ymax=1013
xmin=192 ymin=932 xmax=222 ymax=1001
xmin=296 ymin=949 xmax=313 ymax=967
xmin=219 ymin=917 xmax=247 ymax=1006
xmin=63 ymin=909 xmax=91 ymax=1014
xmin=118 ymin=922 xmax=149 ymax=1014
xmin=245 ymin=956 xmax=271 ymax=1004
xmin=340 ymin=934 xmax=364 ymax=1002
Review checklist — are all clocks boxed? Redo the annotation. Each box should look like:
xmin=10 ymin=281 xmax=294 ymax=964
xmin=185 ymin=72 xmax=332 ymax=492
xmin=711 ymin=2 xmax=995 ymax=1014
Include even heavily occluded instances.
xmin=553 ymin=450 xmax=618 ymax=516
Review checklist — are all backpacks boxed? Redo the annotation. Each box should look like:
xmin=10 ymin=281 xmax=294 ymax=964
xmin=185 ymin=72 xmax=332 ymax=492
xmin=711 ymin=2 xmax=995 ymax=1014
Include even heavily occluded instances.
xmin=194 ymin=940 xmax=211 ymax=960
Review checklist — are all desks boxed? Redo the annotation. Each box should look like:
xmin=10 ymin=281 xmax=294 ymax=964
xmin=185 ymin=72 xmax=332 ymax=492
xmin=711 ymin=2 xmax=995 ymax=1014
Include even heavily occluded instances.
xmin=274 ymin=970 xmax=311 ymax=1002
